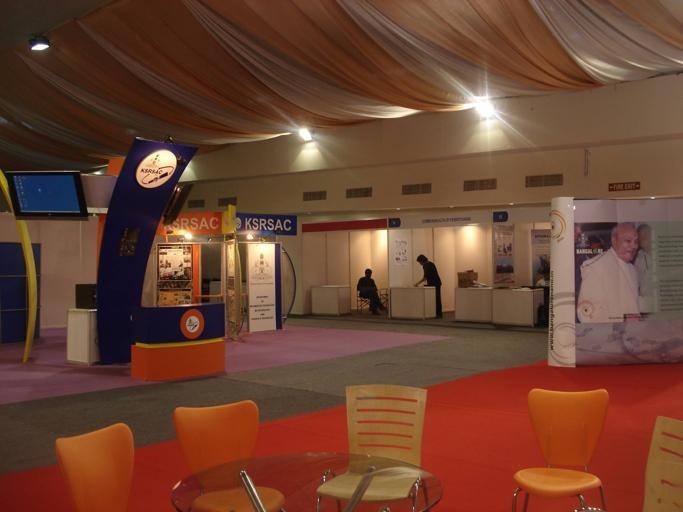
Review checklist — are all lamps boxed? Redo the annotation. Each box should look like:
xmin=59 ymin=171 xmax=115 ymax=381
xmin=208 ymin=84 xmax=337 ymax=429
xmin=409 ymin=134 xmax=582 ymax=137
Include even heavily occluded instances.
xmin=28 ymin=32 xmax=50 ymax=51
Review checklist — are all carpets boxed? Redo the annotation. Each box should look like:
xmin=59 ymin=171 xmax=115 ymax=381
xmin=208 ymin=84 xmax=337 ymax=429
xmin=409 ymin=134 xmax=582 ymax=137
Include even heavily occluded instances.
xmin=2 ymin=358 xmax=663 ymax=511
xmin=2 ymin=320 xmax=455 ymax=406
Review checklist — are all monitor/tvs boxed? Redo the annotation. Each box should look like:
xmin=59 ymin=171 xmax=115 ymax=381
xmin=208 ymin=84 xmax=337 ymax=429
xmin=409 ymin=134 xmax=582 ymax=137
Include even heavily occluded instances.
xmin=163 ymin=182 xmax=193 ymax=224
xmin=76 ymin=284 xmax=97 ymax=309
xmin=3 ymin=169 xmax=89 ymax=217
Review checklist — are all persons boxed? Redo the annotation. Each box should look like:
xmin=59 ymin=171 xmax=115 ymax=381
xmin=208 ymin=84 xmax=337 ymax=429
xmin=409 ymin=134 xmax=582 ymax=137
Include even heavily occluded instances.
xmin=158 ymin=246 xmax=193 ymax=289
xmin=634 ymin=224 xmax=653 ymax=298
xmin=576 ymin=221 xmax=647 ymax=323
xmin=357 ymin=269 xmax=387 ymax=315
xmin=415 ymin=254 xmax=443 ymax=320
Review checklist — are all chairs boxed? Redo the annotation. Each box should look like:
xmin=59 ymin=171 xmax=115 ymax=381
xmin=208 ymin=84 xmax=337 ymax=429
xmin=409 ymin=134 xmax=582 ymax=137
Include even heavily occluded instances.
xmin=642 ymin=415 xmax=683 ymax=512
xmin=512 ymin=388 xmax=610 ymax=512
xmin=315 ymin=384 xmax=433 ymax=512
xmin=169 ymin=400 xmax=287 ymax=512
xmin=55 ymin=422 xmax=135 ymax=512
xmin=356 ymin=287 xmax=388 ymax=314
